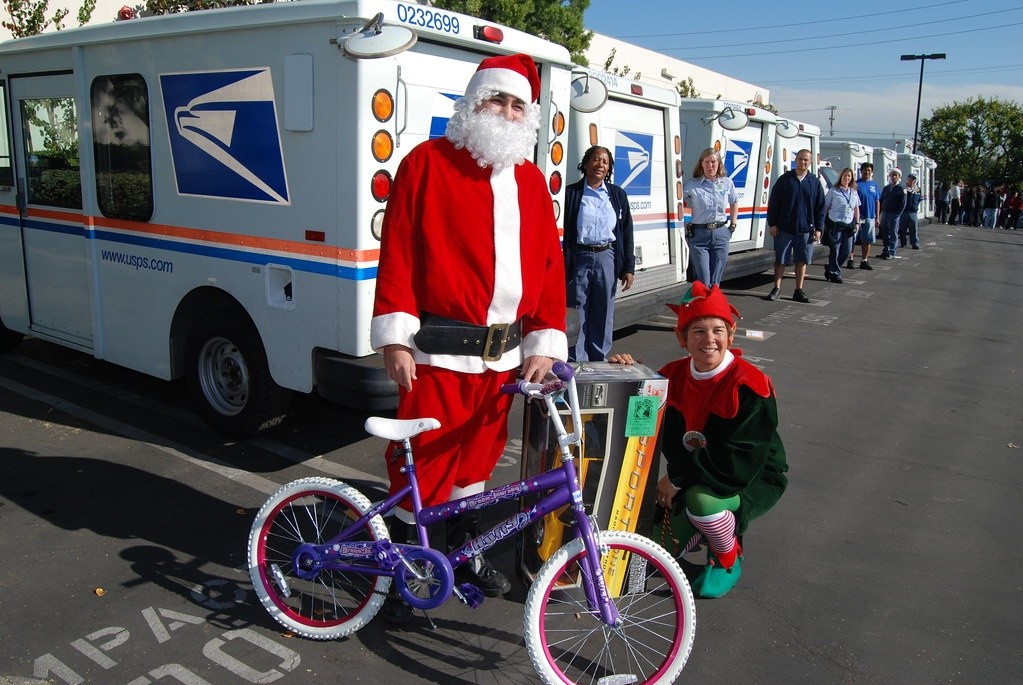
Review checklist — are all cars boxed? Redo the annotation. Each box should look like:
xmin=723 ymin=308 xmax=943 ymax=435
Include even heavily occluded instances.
xmin=532 ymin=413 xmax=603 ymax=572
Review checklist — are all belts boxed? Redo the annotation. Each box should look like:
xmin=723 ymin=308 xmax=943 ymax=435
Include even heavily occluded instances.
xmin=575 ymin=242 xmax=613 ymax=252
xmin=692 ymin=219 xmax=728 ymax=230
xmin=412 ymin=309 xmax=522 ymax=362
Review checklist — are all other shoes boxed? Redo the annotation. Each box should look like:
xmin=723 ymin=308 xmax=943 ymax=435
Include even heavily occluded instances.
xmin=884 ymin=254 xmax=894 ymax=260
xmin=830 ymin=275 xmax=843 ymax=283
xmin=876 ymin=253 xmax=886 ymax=258
xmin=860 ymin=261 xmax=872 ymax=270
xmin=912 ymin=244 xmax=919 ymax=249
xmin=698 ymin=555 xmax=745 ymax=598
xmin=901 ymin=244 xmax=906 ymax=247
xmin=938 ymin=218 xmax=1023 ymax=230
xmin=847 ymin=261 xmax=854 ymax=269
xmin=824 ymin=271 xmax=831 ymax=282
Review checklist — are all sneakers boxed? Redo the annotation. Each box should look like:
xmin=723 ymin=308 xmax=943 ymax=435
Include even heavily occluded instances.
xmin=768 ymin=287 xmax=780 ymax=299
xmin=794 ymin=288 xmax=809 ymax=302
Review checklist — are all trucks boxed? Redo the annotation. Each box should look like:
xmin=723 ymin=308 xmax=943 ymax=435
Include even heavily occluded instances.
xmin=0 ymin=0 xmax=578 ymax=442
xmin=763 ymin=116 xmax=830 ymax=266
xmin=678 ymin=98 xmax=777 ymax=282
xmin=820 ymin=140 xmax=937 ymax=220
xmin=564 ymin=64 xmax=690 ymax=349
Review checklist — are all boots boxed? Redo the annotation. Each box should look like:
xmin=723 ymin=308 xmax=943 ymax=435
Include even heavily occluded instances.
xmin=380 ymin=517 xmax=421 ymax=625
xmin=444 ymin=510 xmax=513 ymax=597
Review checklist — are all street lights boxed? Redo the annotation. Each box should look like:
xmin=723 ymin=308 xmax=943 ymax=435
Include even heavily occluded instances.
xmin=900 ymin=53 xmax=946 ymax=154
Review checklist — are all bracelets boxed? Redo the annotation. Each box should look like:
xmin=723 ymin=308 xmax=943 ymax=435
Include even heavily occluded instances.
xmin=855 ymin=222 xmax=859 ymax=224
xmin=731 ymin=222 xmax=736 ymax=227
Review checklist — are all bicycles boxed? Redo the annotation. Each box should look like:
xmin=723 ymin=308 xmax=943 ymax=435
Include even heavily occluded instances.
xmin=247 ymin=360 xmax=696 ymax=685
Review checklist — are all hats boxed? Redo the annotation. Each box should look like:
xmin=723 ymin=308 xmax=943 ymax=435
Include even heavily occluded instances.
xmin=889 ymin=166 xmax=903 ymax=177
xmin=908 ymin=174 xmax=916 ymax=179
xmin=664 ymin=279 xmax=743 ymax=349
xmin=465 ymin=53 xmax=541 ymax=107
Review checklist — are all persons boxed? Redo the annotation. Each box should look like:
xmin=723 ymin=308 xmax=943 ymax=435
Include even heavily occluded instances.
xmin=684 ymin=148 xmax=739 ymax=289
xmin=767 ymin=149 xmax=826 ymax=301
xmin=563 ymin=146 xmax=635 ymax=362
xmin=935 ymin=179 xmax=1023 ymax=230
xmin=848 ymin=162 xmax=881 ymax=270
xmin=876 ymin=166 xmax=907 ymax=261
xmin=370 ymin=54 xmax=568 ymax=597
xmin=825 ymin=167 xmax=862 ymax=283
xmin=609 ymin=281 xmax=788 ymax=598
xmin=899 ymin=174 xmax=922 ymax=250
